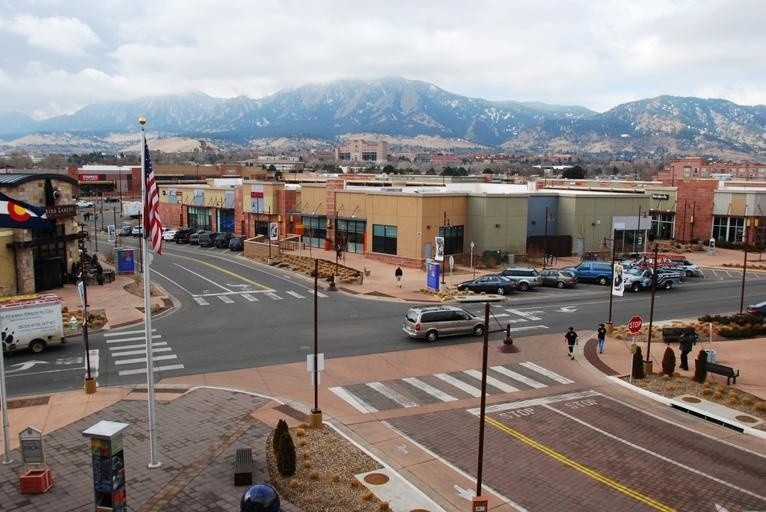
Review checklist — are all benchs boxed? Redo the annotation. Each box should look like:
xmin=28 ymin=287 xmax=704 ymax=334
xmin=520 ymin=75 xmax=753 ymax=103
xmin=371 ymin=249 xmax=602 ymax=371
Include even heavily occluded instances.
xmin=232 ymin=445 xmax=255 ymax=486
xmin=695 ymin=358 xmax=744 ymax=385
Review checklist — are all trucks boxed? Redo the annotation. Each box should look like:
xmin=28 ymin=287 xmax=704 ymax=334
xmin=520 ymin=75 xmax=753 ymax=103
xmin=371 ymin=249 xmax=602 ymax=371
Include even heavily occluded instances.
xmin=0 ymin=290 xmax=65 ymax=358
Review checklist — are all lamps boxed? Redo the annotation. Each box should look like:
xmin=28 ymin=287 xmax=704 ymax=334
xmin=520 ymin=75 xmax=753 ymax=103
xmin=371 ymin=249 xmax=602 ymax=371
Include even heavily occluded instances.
xmin=591 ymin=219 xmax=602 ymax=227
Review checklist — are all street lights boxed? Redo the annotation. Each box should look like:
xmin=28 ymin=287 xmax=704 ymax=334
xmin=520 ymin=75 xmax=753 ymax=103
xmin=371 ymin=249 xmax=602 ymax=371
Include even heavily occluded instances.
xmin=260 ymin=205 xmax=272 ymax=266
xmin=92 ymin=203 xmax=102 ymax=253
xmin=438 ymin=209 xmax=451 ymax=284
xmin=636 ymin=243 xmax=660 ymax=375
xmin=175 ymin=193 xmax=183 ymax=227
xmin=112 ymin=205 xmax=122 ymax=250
xmin=78 ymin=222 xmax=96 ymax=394
xmin=731 ymin=227 xmax=748 ymax=318
xmin=542 ymin=206 xmax=554 ymax=270
xmin=634 ymin=204 xmax=647 ymax=254
xmin=325 ymin=211 xmax=340 ymax=284
xmin=470 ymin=302 xmax=521 ymax=512
xmin=215 ymin=195 xmax=222 ymax=233
xmin=598 ymin=234 xmax=618 ymax=334
xmin=308 ymin=258 xmax=340 ymax=430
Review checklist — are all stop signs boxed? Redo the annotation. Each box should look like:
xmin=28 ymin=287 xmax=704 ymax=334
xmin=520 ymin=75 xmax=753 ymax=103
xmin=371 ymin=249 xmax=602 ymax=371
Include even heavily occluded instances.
xmin=627 ymin=315 xmax=643 ymax=336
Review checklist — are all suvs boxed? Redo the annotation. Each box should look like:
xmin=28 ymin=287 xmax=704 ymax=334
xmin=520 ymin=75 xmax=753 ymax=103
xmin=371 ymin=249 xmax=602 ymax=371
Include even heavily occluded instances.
xmin=402 ymin=304 xmax=486 ymax=343
xmin=495 ymin=266 xmax=544 ymax=293
xmin=562 ymin=260 xmax=613 ymax=287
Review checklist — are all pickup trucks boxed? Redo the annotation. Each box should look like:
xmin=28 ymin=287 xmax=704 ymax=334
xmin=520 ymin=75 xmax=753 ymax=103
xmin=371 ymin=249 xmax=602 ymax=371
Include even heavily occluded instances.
xmin=620 ymin=256 xmax=702 ymax=295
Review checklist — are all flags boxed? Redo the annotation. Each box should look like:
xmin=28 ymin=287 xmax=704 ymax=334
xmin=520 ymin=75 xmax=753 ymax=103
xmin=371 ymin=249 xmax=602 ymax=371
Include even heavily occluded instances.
xmin=141 ymin=130 xmax=163 ymax=254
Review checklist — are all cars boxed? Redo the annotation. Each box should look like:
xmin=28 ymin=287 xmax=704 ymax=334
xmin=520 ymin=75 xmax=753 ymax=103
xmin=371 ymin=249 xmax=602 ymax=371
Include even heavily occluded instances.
xmin=456 ymin=271 xmax=519 ymax=296
xmin=132 ymin=225 xmax=144 ymax=237
xmin=538 ymin=267 xmax=580 ymax=291
xmin=746 ymin=299 xmax=766 ymax=318
xmin=160 ymin=226 xmax=246 ymax=253
xmin=119 ymin=224 xmax=131 ymax=237
xmin=76 ymin=199 xmax=93 ymax=209
xmin=103 ymin=195 xmax=120 ymax=204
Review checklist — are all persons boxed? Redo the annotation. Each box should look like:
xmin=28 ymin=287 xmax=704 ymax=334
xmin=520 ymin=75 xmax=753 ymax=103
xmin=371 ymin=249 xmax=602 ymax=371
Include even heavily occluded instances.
xmin=395 ymin=267 xmax=402 ymax=288
xmin=598 ymin=324 xmax=606 ymax=354
xmin=77 ymin=266 xmax=82 ymax=288
xmin=83 ymin=212 xmax=98 ymax=222
xmin=679 ymin=331 xmax=691 ymax=371
xmin=565 ymin=327 xmax=578 ymax=360
xmin=337 ymin=242 xmax=342 ymax=261
